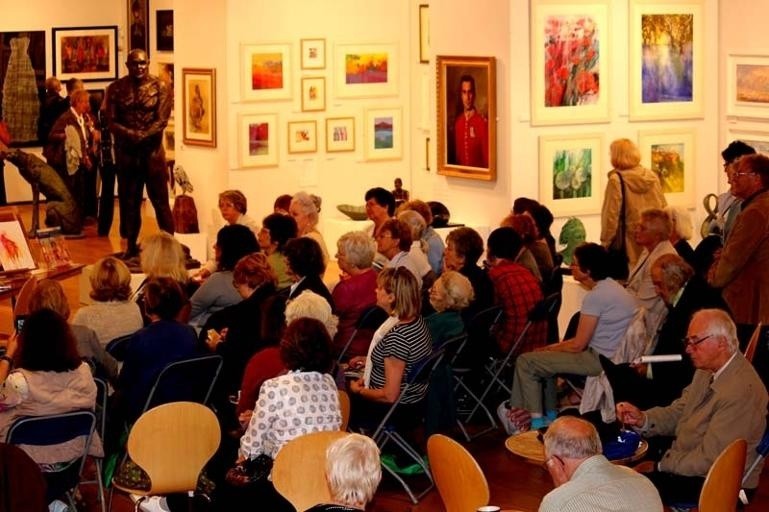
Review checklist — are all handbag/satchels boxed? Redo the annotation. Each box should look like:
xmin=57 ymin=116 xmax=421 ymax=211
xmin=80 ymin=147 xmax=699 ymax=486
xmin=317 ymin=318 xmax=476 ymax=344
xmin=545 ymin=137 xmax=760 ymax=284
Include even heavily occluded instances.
xmin=605 ymin=241 xmax=630 ymax=281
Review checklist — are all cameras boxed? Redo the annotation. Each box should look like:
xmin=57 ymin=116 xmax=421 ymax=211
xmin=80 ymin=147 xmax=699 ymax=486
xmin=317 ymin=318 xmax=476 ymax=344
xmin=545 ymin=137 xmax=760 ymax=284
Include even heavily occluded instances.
xmin=14 ymin=315 xmax=27 ymax=332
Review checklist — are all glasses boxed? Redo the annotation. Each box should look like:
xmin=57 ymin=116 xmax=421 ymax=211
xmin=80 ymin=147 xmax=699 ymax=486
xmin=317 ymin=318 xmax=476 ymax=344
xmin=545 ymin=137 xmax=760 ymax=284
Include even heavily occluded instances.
xmin=735 ymin=171 xmax=756 ymax=177
xmin=681 ymin=333 xmax=716 ymax=347
xmin=541 ymin=454 xmax=565 ymax=471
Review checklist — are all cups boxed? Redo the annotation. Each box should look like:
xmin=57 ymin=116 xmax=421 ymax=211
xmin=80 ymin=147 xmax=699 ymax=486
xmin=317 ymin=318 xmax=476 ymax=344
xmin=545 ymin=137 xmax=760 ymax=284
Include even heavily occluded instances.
xmin=477 ymin=505 xmax=501 ymax=512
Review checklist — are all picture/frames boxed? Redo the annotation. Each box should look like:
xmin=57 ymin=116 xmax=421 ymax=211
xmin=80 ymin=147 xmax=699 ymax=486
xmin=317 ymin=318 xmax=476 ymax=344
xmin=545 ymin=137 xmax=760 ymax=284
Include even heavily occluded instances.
xmin=425 ymin=138 xmax=431 ymax=171
xmin=156 ymin=60 xmax=175 ymax=121
xmin=236 ymin=36 xmax=404 ymax=171
xmin=638 ymin=125 xmax=698 ymax=212
xmin=126 ymin=0 xmax=151 ymax=64
xmin=725 ymin=50 xmax=769 ymax=120
xmin=626 ymin=0 xmax=707 ymax=124
xmin=537 ymin=133 xmax=609 ymax=219
xmin=419 ymin=3 xmax=430 ymax=64
xmin=435 ymin=54 xmax=498 ymax=182
xmin=51 ymin=26 xmax=120 ymax=83
xmin=529 ymin=1 xmax=613 ymax=129
xmin=726 ymin=125 xmax=769 ymax=160
xmin=181 ymin=67 xmax=217 ymax=144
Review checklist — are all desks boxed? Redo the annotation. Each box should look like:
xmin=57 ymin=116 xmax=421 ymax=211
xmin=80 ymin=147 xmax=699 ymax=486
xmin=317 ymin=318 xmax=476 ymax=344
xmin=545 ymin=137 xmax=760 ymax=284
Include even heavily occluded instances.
xmin=2 ymin=262 xmax=82 ymax=296
xmin=505 ymin=426 xmax=651 ymax=470
xmin=78 ymin=263 xmax=205 ymax=307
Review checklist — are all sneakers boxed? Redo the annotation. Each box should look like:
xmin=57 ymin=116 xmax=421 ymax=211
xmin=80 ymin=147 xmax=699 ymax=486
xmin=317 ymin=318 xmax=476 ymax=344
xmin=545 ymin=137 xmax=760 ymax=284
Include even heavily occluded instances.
xmin=48 ymin=499 xmax=70 ymax=512
xmin=129 ymin=493 xmax=171 ymax=512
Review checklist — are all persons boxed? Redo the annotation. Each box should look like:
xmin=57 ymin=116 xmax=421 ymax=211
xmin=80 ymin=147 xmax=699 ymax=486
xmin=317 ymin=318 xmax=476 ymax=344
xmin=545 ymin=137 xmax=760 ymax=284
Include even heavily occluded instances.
xmin=99 ymin=48 xmax=176 ymax=258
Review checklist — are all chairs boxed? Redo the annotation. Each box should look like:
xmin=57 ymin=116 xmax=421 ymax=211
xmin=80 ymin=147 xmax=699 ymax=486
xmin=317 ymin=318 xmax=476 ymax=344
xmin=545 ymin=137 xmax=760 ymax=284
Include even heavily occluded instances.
xmin=697 ymin=430 xmax=769 ymax=512
xmin=332 ymin=257 xmax=590 ymax=512
xmin=743 ymin=319 xmax=764 ymax=362
xmin=5 ymin=331 xmax=223 ymax=511
